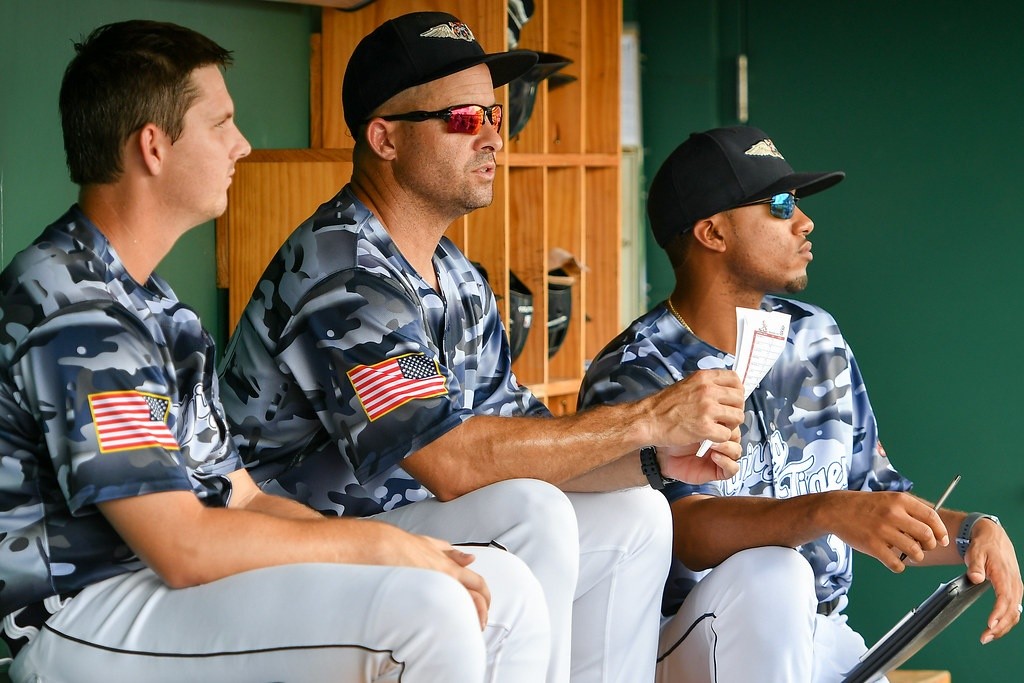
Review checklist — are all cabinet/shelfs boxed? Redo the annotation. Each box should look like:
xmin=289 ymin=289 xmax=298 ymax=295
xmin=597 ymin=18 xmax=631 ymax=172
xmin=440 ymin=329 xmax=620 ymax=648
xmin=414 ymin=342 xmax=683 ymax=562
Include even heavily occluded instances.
xmin=217 ymin=1 xmax=623 ymax=418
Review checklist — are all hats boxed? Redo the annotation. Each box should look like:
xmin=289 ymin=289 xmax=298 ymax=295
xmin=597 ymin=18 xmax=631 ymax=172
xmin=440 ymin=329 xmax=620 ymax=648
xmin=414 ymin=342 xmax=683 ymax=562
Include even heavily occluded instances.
xmin=343 ymin=11 xmax=540 ymax=143
xmin=647 ymin=126 xmax=845 ymax=248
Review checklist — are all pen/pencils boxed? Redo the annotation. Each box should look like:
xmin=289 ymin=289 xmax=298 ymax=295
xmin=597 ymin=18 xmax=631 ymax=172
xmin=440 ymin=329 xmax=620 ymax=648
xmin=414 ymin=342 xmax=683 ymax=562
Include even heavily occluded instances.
xmin=899 ymin=474 xmax=962 ymax=561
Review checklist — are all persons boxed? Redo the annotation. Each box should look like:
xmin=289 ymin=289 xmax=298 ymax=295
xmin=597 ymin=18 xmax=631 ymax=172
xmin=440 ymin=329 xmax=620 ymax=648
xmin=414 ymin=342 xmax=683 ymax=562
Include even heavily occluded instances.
xmin=576 ymin=126 xmax=1024 ymax=683
xmin=0 ymin=21 xmax=550 ymax=683
xmin=218 ymin=12 xmax=745 ymax=683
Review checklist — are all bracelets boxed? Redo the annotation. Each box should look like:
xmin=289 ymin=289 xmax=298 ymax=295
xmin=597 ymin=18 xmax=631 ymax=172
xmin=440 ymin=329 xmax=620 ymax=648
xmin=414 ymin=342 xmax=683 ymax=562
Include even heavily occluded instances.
xmin=639 ymin=445 xmax=678 ymax=490
xmin=955 ymin=512 xmax=1002 ymax=561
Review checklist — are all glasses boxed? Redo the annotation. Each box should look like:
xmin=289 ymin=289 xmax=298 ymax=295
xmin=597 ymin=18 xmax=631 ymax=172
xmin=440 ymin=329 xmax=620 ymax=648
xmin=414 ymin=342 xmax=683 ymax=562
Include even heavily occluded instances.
xmin=365 ymin=102 xmax=503 ymax=135
xmin=682 ymin=192 xmax=800 ymax=234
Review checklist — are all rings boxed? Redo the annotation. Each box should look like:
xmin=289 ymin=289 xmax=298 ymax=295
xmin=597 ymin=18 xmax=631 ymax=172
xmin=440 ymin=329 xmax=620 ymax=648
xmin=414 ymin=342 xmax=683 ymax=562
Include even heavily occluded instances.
xmin=1018 ymin=604 xmax=1022 ymax=613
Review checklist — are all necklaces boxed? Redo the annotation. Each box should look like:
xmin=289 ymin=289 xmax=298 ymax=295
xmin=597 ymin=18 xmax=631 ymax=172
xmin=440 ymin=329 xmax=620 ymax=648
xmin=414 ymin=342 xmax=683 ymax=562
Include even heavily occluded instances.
xmin=667 ymin=297 xmax=697 ymax=337
